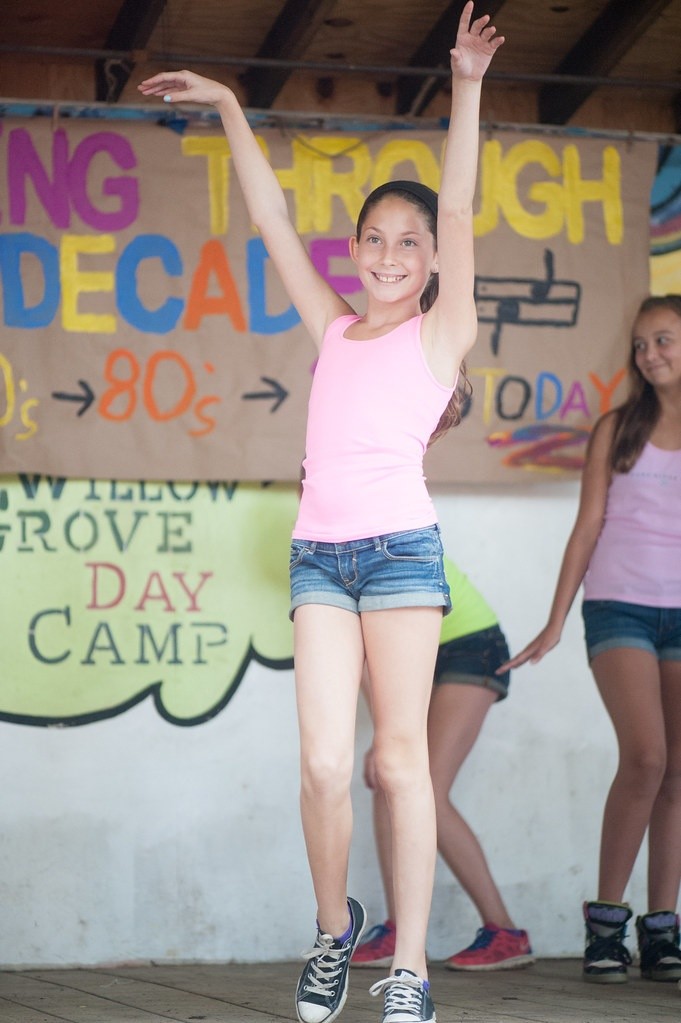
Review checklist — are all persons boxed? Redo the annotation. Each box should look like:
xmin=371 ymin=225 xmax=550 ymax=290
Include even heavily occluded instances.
xmin=137 ymin=0 xmax=506 ymax=1023
xmin=299 ymin=450 xmax=534 ymax=974
xmin=494 ymin=293 xmax=681 ymax=983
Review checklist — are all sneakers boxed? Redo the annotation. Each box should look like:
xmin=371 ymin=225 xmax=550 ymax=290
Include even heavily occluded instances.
xmin=349 ymin=921 xmax=429 ymax=967
xmin=368 ymin=969 xmax=437 ymax=1023
xmin=295 ymin=896 xmax=368 ymax=1023
xmin=635 ymin=910 xmax=681 ymax=980
xmin=582 ymin=901 xmax=633 ymax=983
xmin=443 ymin=923 xmax=534 ymax=972
